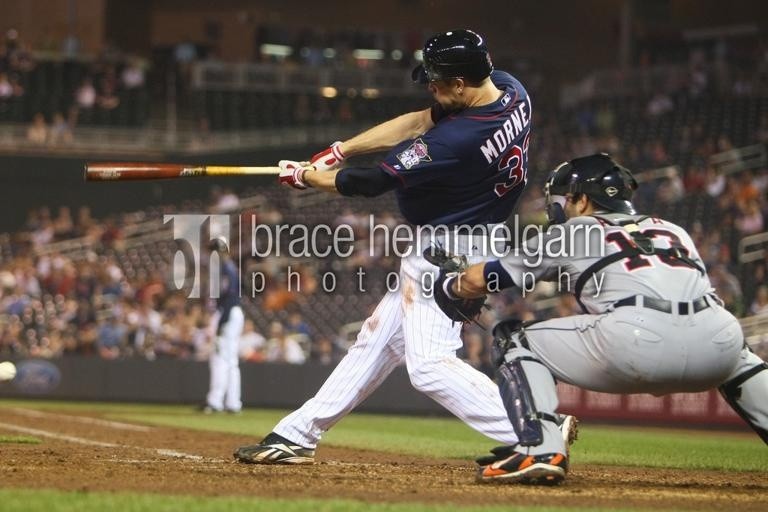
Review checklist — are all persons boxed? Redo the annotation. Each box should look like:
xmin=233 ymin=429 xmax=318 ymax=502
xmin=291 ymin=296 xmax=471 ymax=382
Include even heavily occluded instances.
xmin=1 ymin=15 xmax=768 ymax=416
xmin=432 ymin=151 xmax=768 ymax=485
xmin=235 ymin=29 xmax=578 ymax=467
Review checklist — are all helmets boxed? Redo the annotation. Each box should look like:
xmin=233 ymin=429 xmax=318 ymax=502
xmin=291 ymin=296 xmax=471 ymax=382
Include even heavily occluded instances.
xmin=544 ymin=154 xmax=638 ymax=224
xmin=412 ymin=30 xmax=494 ymax=83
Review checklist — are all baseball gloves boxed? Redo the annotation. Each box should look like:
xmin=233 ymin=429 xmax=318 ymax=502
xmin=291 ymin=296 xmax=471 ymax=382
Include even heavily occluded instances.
xmin=430 ymin=246 xmax=485 ymax=326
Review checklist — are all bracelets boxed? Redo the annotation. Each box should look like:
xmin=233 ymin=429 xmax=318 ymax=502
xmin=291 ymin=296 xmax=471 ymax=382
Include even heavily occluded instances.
xmin=302 ymin=168 xmax=320 ymax=190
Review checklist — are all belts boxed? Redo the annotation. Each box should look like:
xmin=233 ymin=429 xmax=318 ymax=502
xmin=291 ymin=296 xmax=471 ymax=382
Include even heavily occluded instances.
xmin=613 ymin=295 xmax=719 ymax=315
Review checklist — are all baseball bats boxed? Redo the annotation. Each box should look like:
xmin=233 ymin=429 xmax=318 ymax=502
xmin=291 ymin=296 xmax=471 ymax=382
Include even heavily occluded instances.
xmin=83 ymin=160 xmax=303 ymax=182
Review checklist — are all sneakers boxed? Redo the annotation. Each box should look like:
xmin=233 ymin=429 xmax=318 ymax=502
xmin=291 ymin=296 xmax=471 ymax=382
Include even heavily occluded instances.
xmin=479 ymin=453 xmax=566 ymax=486
xmin=233 ymin=433 xmax=315 ymax=464
xmin=476 ymin=411 xmax=579 ymax=469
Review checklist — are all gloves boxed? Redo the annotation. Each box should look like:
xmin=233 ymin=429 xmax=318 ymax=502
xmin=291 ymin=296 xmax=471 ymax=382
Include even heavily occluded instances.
xmin=310 ymin=141 xmax=347 ymax=171
xmin=279 ymin=160 xmax=315 ymax=190
